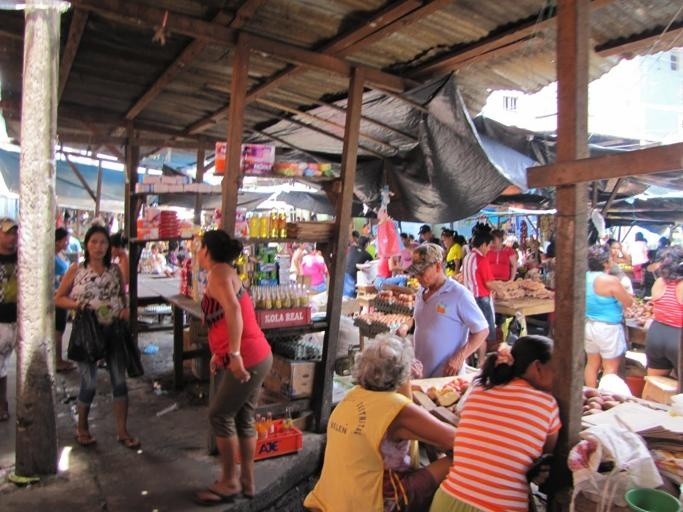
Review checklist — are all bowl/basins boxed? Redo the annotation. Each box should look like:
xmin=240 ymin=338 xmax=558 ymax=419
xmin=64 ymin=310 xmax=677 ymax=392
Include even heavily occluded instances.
xmin=625 ymin=487 xmax=683 ymax=512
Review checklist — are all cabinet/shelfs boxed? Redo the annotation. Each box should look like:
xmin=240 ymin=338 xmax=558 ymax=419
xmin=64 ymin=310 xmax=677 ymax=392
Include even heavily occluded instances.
xmin=126 ymin=182 xmax=338 ymax=457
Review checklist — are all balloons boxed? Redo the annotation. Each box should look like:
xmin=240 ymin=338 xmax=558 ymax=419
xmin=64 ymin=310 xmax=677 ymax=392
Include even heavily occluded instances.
xmin=272 ymin=161 xmax=333 ymax=177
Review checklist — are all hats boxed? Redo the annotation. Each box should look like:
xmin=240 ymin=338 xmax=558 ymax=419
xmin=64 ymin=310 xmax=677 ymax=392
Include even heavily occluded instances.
xmin=403 ymin=243 xmax=444 ymax=275
xmin=0 ymin=217 xmax=18 ymax=233
xmin=418 ymin=225 xmax=431 ymax=235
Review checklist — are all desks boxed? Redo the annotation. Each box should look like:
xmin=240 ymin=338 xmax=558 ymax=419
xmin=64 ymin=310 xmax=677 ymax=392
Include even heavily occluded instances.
xmin=491 ymin=290 xmax=554 ymax=335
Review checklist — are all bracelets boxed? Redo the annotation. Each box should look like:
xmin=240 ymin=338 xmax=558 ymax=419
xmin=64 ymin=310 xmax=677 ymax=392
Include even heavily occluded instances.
xmin=231 ymin=351 xmax=240 ymax=358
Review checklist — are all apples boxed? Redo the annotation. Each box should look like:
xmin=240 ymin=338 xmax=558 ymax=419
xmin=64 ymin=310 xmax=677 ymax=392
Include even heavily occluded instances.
xmin=448 ymin=376 xmax=470 ymax=395
xmin=581 ymin=389 xmax=623 ymax=414
xmin=379 ymin=288 xmax=413 ymax=309
xmin=358 ymin=309 xmax=412 ymax=326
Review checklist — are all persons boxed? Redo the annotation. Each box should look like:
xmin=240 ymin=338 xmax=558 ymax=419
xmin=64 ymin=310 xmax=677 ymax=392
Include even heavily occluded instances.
xmin=584 ymin=222 xmax=683 ymax=389
xmin=193 ymin=230 xmax=273 ymax=504
xmin=289 ymin=223 xmax=556 ymax=379
xmin=0 ymin=217 xmax=18 ymax=422
xmin=302 ymin=334 xmax=456 ymax=512
xmin=54 ymin=225 xmax=141 ymax=450
xmin=429 ymin=336 xmax=563 ymax=512
xmin=56 ymin=227 xmax=179 ymax=372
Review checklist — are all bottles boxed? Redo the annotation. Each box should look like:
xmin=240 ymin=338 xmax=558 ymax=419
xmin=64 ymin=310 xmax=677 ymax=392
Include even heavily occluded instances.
xmin=253 ymin=262 xmax=265 ymax=285
xmin=256 ymin=412 xmax=274 ymax=439
xmin=250 ymin=207 xmax=287 ymax=240
xmin=250 ymin=283 xmax=310 ymax=309
xmin=284 ymin=407 xmax=293 ymax=431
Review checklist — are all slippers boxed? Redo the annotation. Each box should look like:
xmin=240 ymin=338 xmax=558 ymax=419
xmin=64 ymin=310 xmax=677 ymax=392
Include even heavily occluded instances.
xmin=75 ymin=434 xmax=97 ymax=447
xmin=56 ymin=362 xmax=74 ymax=371
xmin=117 ymin=435 xmax=142 ymax=449
xmin=191 ymin=477 xmax=254 ymax=506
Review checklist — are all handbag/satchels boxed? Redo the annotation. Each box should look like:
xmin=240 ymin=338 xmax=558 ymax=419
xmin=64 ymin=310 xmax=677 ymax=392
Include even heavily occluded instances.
xmin=382 ymin=439 xmax=416 ymax=473
xmin=567 ymin=424 xmax=664 ymax=507
